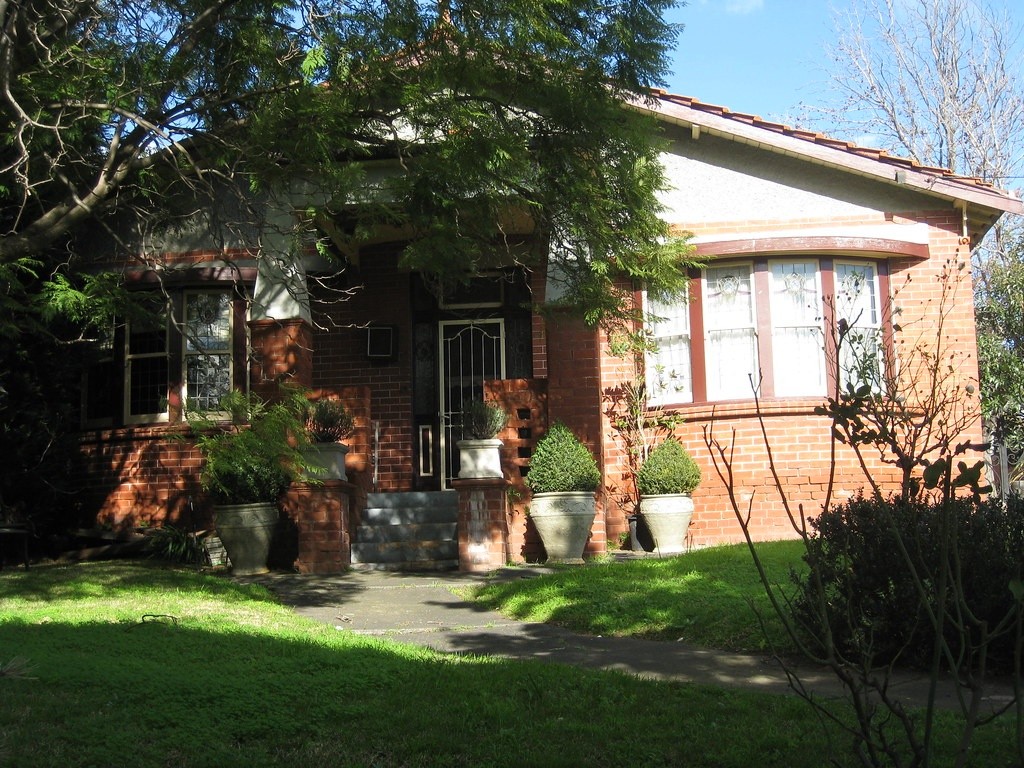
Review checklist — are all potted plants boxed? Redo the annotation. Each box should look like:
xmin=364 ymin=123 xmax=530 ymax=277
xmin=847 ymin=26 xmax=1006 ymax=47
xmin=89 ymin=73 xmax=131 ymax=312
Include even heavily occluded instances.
xmin=635 ymin=440 xmax=703 ymax=554
xmin=158 ymin=367 xmax=328 ymax=576
xmin=523 ymin=418 xmax=601 ymax=566
xmin=455 ymin=397 xmax=508 ymax=478
xmin=614 ymin=357 xmax=686 ymax=552
xmin=297 ymin=398 xmax=356 ymax=482
